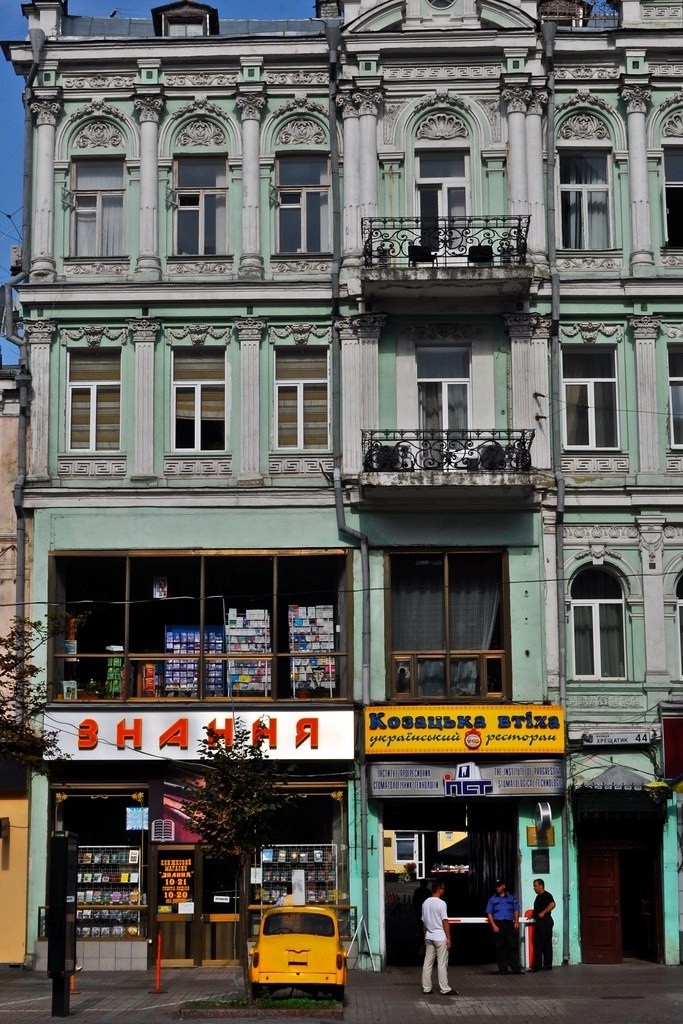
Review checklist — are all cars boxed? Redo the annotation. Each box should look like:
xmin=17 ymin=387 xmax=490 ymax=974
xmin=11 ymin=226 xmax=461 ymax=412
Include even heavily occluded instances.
xmin=249 ymin=905 xmax=349 ymax=1004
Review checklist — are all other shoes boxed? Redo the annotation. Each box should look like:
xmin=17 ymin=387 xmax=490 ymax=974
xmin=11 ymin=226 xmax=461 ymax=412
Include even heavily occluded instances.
xmin=543 ymin=967 xmax=553 ymax=971
xmin=499 ymin=970 xmax=509 ymax=975
xmin=441 ymin=989 xmax=459 ymax=995
xmin=512 ymin=970 xmax=526 ymax=975
xmin=527 ymin=967 xmax=542 ymax=973
xmin=423 ymin=990 xmax=434 ymax=994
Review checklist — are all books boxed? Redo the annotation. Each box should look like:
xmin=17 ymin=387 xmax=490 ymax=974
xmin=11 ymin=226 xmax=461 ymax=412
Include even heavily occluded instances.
xmin=75 ymin=849 xmax=139 ymax=938
xmin=263 ymin=848 xmax=335 ymax=902
xmin=62 ymin=680 xmax=77 ymax=699
xmin=167 ymin=631 xmax=223 ymax=689
xmin=141 ymin=662 xmax=164 ymax=698
xmin=224 ymin=608 xmax=272 ymax=690
xmin=105 ymin=658 xmax=121 ymax=693
xmin=153 ymin=577 xmax=167 ymax=599
xmin=287 ymin=605 xmax=336 ymax=688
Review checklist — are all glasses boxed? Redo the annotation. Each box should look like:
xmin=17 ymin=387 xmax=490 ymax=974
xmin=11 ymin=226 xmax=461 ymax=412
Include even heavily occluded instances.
xmin=440 ymin=888 xmax=445 ymax=890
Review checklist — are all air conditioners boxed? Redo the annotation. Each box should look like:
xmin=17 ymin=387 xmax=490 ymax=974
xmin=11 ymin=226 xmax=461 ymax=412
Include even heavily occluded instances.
xmin=10 ymin=245 xmax=21 ymax=275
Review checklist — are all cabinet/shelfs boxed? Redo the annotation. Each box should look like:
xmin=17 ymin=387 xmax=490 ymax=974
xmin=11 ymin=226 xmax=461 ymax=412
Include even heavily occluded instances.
xmin=164 ymin=623 xmax=226 ymax=697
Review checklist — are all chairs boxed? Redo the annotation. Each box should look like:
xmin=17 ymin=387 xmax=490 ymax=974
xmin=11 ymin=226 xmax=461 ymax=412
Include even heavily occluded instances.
xmin=378 ymin=446 xmax=399 ymax=471
xmin=480 ymin=445 xmax=505 ymax=470
xmin=467 ymin=245 xmax=494 ymax=267
xmin=408 ymin=246 xmax=439 ymax=268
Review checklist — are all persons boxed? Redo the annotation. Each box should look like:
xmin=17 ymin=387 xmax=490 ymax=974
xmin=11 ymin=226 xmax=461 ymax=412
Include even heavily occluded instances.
xmin=486 ymin=879 xmax=526 ymax=975
xmin=527 ymin=879 xmax=556 ymax=972
xmin=420 ymin=880 xmax=459 ymax=995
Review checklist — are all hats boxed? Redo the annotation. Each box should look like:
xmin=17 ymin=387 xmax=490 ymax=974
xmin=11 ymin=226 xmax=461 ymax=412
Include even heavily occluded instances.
xmin=495 ymin=879 xmax=505 ymax=887
